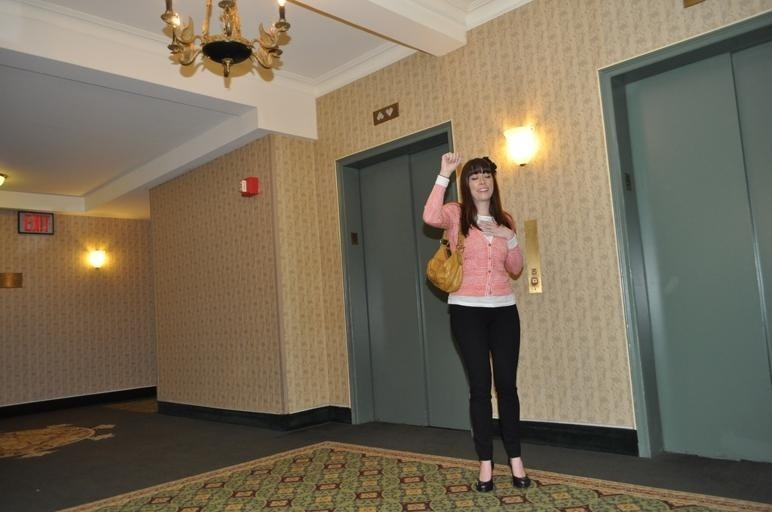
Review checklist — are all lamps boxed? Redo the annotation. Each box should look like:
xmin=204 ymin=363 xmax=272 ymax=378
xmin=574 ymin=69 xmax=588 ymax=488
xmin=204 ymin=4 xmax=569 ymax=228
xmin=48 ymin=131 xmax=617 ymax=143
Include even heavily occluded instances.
xmin=160 ymin=0 xmax=291 ymax=78
xmin=0 ymin=173 xmax=8 ymax=187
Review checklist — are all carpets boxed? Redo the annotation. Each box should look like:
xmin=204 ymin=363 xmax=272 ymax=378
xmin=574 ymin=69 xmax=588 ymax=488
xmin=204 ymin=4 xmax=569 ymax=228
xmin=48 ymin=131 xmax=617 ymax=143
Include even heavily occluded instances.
xmin=58 ymin=441 xmax=772 ymax=512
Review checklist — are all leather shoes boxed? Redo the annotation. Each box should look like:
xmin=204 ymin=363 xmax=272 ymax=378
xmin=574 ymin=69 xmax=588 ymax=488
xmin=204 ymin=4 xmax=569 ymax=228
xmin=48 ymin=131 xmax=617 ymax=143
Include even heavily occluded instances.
xmin=477 ymin=461 xmax=494 ymax=492
xmin=508 ymin=457 xmax=530 ymax=487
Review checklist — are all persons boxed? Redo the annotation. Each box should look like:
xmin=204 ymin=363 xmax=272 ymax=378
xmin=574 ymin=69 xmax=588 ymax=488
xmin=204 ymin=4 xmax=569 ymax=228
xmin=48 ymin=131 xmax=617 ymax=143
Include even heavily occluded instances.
xmin=422 ymin=150 xmax=530 ymax=495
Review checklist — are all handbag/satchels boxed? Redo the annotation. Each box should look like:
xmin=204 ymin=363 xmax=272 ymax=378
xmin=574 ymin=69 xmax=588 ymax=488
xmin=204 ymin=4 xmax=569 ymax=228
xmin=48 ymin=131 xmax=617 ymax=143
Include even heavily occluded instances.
xmin=426 ymin=236 xmax=464 ymax=293
